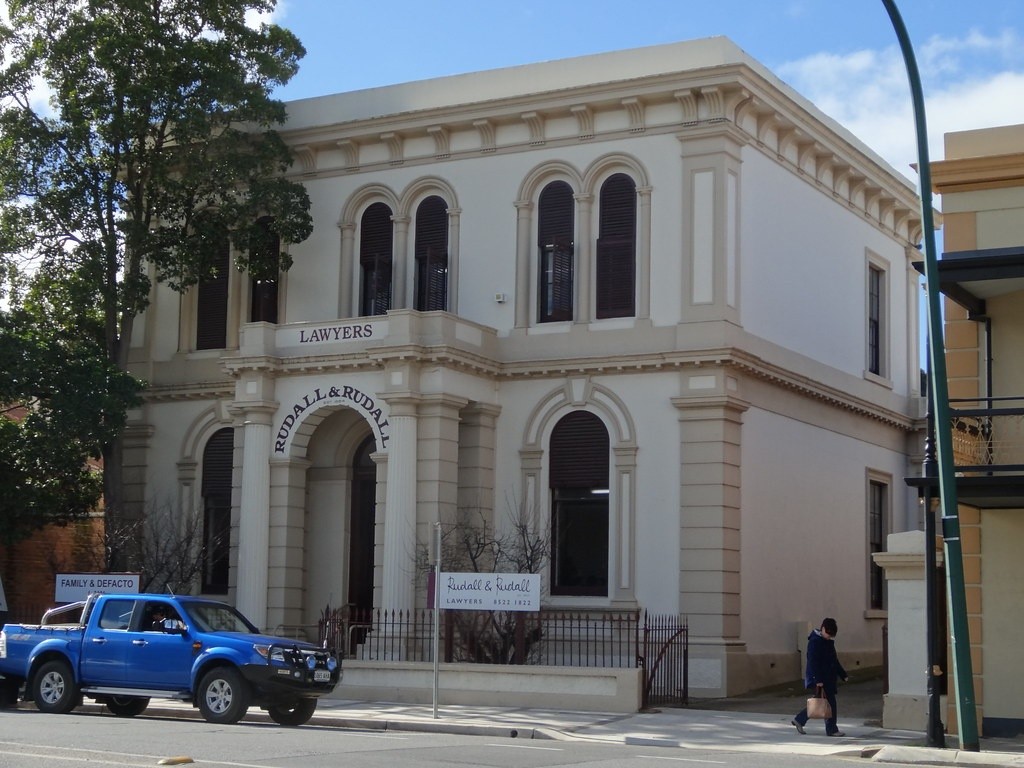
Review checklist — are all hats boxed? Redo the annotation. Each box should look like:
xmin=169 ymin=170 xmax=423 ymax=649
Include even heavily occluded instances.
xmin=823 ymin=618 xmax=837 ymax=636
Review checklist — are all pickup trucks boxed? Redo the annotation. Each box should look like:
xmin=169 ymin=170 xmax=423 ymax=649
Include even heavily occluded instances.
xmin=0 ymin=590 xmax=343 ymax=728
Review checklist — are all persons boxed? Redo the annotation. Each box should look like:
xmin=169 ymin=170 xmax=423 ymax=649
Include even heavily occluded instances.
xmin=150 ymin=609 xmax=187 ymax=630
xmin=791 ymin=618 xmax=848 ymax=736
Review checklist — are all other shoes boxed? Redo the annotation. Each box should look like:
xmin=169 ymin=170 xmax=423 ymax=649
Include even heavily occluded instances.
xmin=831 ymin=731 xmax=846 ymax=737
xmin=792 ymin=719 xmax=806 ymax=735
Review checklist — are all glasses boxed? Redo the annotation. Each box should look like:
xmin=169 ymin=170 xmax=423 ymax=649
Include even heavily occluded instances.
xmin=155 ymin=613 xmax=168 ymax=617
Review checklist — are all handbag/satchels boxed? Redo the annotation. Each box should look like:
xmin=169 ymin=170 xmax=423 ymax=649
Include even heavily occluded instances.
xmin=806 ymin=686 xmax=831 ymax=719
